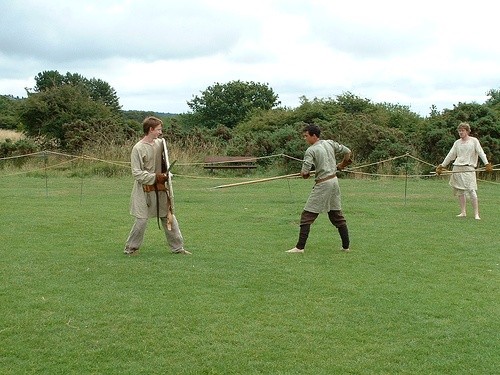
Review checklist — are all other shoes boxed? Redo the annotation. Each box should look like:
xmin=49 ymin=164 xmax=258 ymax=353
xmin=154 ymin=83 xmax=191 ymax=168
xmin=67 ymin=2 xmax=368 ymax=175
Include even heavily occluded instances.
xmin=340 ymin=246 xmax=351 ymax=251
xmin=286 ymin=247 xmax=306 ymax=253
xmin=474 ymin=215 xmax=481 ymax=220
xmin=175 ymin=248 xmax=192 ymax=255
xmin=456 ymin=212 xmax=467 ymax=217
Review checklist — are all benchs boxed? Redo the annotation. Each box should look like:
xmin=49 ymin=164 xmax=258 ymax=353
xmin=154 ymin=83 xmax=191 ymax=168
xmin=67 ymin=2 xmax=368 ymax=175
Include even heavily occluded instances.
xmin=204 ymin=156 xmax=257 ymax=173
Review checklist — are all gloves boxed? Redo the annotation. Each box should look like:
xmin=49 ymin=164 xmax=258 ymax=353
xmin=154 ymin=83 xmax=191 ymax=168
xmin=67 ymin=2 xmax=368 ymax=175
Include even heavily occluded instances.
xmin=301 ymin=169 xmax=310 ymax=179
xmin=485 ymin=164 xmax=493 ymax=174
xmin=156 ymin=172 xmax=168 ymax=184
xmin=337 ymin=152 xmax=354 ymax=170
xmin=435 ymin=165 xmax=443 ymax=176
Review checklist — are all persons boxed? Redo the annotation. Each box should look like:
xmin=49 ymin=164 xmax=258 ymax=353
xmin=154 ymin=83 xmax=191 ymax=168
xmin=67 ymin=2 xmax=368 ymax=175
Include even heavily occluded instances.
xmin=285 ymin=124 xmax=352 ymax=253
xmin=123 ymin=116 xmax=192 ymax=256
xmin=436 ymin=123 xmax=492 ymax=220
